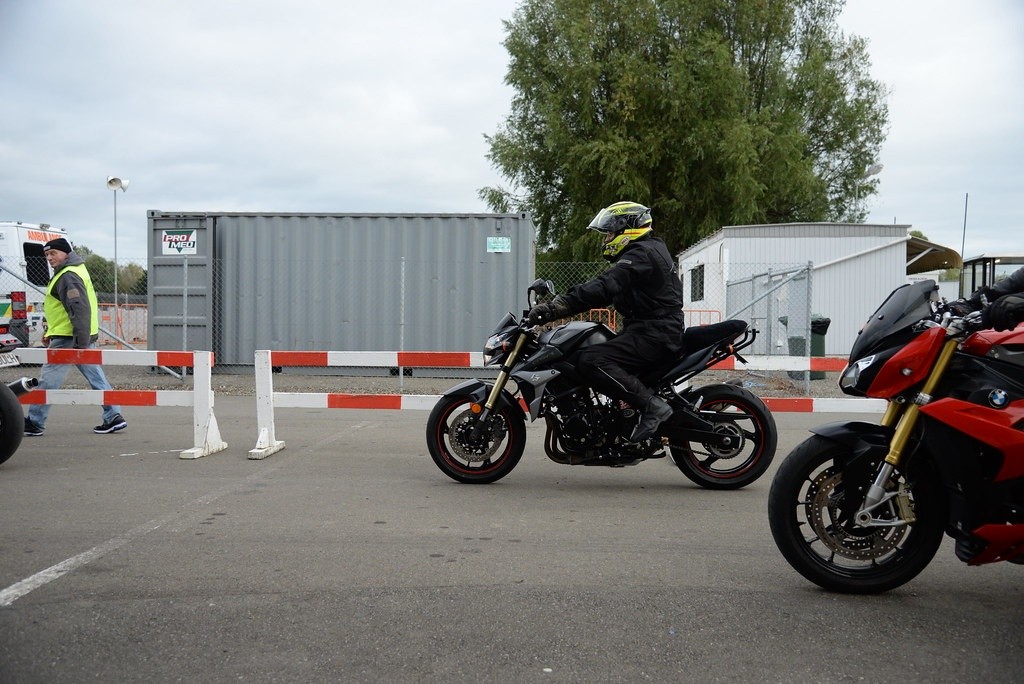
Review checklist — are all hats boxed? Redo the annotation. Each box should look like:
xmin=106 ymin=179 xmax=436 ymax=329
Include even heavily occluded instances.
xmin=43 ymin=238 xmax=72 ymax=254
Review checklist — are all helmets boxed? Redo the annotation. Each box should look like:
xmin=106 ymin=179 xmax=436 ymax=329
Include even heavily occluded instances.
xmin=586 ymin=201 xmax=653 ymax=261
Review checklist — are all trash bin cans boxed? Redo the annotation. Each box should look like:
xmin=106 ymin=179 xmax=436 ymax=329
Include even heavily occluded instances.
xmin=778 ymin=316 xmax=831 ymax=380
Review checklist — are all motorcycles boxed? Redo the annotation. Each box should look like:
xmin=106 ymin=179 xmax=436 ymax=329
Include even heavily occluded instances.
xmin=766 ymin=279 xmax=1024 ymax=596
xmin=1 ymin=318 xmax=40 ymax=467
xmin=426 ymin=276 xmax=778 ymax=492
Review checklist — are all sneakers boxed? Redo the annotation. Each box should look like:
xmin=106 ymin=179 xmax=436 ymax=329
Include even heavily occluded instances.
xmin=24 ymin=417 xmax=43 ymax=436
xmin=630 ymin=397 xmax=674 ymax=442
xmin=94 ymin=415 xmax=127 ymax=434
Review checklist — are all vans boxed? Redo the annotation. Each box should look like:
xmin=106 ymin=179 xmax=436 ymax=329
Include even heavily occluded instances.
xmin=1 ymin=220 xmax=74 ymax=365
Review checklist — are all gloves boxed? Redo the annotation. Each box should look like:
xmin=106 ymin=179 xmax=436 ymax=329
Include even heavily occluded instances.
xmin=949 ymin=297 xmax=973 ymax=317
xmin=528 ymin=302 xmax=552 ymax=325
xmin=982 ymin=293 xmax=1024 ymax=331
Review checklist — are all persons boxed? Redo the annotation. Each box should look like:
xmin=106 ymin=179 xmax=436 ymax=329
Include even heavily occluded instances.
xmin=529 ymin=201 xmax=686 ymax=442
xmin=946 ymin=264 xmax=1024 ymax=332
xmin=21 ymin=237 xmax=127 ymax=437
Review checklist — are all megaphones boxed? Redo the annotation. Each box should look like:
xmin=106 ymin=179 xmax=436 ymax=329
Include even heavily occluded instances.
xmin=107 ymin=176 xmax=130 ymax=193
